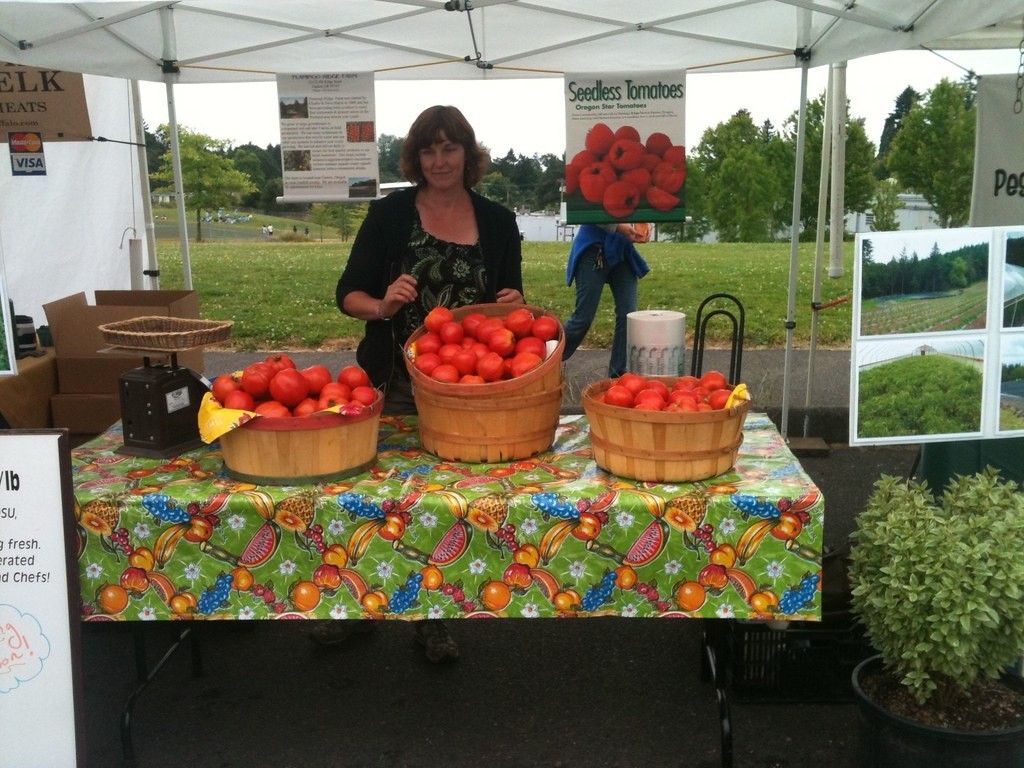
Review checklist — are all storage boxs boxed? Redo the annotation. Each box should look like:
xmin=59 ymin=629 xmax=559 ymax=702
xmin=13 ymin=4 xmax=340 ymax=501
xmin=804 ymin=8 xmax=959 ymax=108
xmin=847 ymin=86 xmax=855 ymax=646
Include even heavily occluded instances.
xmin=717 ymin=609 xmax=865 ymax=705
xmin=43 ymin=290 xmax=202 ymax=435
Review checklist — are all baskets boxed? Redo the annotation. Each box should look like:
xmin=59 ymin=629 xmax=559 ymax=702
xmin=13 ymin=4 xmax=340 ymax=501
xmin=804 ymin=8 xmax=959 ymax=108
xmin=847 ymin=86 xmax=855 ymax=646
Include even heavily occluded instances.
xmin=402 ymin=303 xmax=567 ymax=463
xmin=218 ymin=388 xmax=385 ymax=486
xmin=98 ymin=316 xmax=234 ymax=352
xmin=582 ymin=374 xmax=750 ymax=483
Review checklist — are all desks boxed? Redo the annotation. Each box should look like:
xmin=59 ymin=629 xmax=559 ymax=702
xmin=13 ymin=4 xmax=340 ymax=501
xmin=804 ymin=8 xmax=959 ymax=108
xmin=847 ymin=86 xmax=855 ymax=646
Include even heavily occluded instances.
xmin=0 ymin=344 xmax=57 ymax=428
xmin=71 ymin=410 xmax=827 ymax=768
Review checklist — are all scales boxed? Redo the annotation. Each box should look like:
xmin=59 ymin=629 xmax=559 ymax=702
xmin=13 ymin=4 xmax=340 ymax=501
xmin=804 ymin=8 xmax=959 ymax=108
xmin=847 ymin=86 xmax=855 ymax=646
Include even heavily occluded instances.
xmin=95 ymin=345 xmax=218 ymax=458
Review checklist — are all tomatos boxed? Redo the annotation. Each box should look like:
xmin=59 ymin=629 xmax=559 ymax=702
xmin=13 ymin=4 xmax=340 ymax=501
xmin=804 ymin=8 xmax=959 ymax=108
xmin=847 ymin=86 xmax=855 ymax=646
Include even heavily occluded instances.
xmin=594 ymin=372 xmax=733 ymax=414
xmin=413 ymin=306 xmax=559 ymax=384
xmin=565 ymin=122 xmax=686 ymax=218
xmin=212 ymin=352 xmax=377 ymax=417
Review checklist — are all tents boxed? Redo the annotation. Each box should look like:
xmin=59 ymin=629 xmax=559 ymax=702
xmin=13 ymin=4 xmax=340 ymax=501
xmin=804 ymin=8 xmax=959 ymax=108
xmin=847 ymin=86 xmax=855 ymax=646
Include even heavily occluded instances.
xmin=1 ymin=0 xmax=1023 ymax=451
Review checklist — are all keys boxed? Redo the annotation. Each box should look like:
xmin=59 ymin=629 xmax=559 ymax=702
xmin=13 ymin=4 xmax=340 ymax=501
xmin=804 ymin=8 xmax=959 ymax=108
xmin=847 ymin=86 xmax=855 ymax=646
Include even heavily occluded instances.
xmin=592 ymin=249 xmax=604 ymax=271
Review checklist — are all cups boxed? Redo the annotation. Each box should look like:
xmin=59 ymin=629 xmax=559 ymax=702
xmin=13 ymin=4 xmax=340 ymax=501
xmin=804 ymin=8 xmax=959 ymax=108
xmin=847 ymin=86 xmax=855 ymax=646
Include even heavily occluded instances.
xmin=14 ymin=315 xmax=36 ymax=352
xmin=36 ymin=328 xmax=54 ymax=347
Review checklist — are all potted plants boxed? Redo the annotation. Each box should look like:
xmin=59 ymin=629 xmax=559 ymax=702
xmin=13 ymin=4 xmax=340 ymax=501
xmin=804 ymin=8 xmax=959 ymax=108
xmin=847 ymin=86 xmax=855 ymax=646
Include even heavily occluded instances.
xmin=848 ymin=465 xmax=1024 ymax=768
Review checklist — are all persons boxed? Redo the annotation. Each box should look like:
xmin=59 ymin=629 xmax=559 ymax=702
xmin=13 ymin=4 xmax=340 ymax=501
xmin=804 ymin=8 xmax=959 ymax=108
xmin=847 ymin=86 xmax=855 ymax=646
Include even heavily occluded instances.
xmin=293 ymin=225 xmax=297 ymax=233
xmin=199 ymin=207 xmax=253 ymax=224
xmin=262 ymin=224 xmax=273 ymax=238
xmin=305 ymin=226 xmax=310 ymax=238
xmin=336 ymin=105 xmax=526 ymax=415
xmin=562 ymin=223 xmax=650 ymax=378
